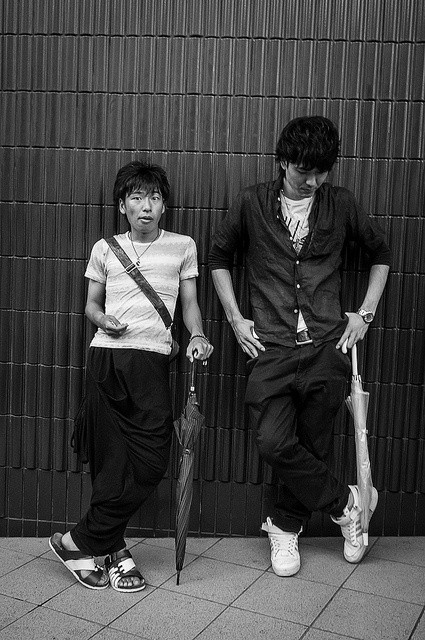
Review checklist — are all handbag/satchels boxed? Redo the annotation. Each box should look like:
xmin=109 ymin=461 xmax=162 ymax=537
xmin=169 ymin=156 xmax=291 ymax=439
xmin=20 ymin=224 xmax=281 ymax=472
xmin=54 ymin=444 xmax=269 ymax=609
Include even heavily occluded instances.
xmin=73 ymin=398 xmax=88 ymax=445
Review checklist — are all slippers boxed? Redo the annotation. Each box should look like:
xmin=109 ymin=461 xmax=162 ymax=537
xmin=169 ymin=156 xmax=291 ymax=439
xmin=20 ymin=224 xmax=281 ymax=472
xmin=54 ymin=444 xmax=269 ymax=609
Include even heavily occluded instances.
xmin=48 ymin=531 xmax=110 ymax=590
xmin=105 ymin=549 xmax=146 ymax=593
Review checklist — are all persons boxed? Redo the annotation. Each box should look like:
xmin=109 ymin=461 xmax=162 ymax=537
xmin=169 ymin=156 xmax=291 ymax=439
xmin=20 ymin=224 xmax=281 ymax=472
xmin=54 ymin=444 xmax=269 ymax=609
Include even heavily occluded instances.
xmin=207 ymin=116 xmax=393 ymax=576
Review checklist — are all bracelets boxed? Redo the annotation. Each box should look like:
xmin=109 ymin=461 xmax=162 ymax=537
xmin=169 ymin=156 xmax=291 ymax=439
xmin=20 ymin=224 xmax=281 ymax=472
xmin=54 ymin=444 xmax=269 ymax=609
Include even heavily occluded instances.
xmin=53 ymin=161 xmax=217 ymax=590
xmin=189 ymin=334 xmax=207 ymax=341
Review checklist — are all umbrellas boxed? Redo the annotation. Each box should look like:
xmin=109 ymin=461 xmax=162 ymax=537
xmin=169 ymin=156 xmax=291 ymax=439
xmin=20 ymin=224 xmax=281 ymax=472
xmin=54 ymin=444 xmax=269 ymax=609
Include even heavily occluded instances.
xmin=173 ymin=348 xmax=207 ymax=584
xmin=341 ymin=338 xmax=372 ymax=547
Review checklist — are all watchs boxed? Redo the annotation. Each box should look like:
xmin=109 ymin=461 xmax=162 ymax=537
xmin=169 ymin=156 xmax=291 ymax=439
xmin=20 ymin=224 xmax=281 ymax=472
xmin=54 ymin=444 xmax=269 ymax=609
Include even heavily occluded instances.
xmin=356 ymin=307 xmax=376 ymax=325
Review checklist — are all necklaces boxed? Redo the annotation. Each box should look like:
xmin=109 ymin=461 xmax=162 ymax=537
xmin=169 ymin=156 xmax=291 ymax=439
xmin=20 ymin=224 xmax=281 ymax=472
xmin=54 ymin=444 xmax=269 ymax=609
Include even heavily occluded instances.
xmin=281 ymin=187 xmax=315 ymax=238
xmin=129 ymin=229 xmax=160 ymax=266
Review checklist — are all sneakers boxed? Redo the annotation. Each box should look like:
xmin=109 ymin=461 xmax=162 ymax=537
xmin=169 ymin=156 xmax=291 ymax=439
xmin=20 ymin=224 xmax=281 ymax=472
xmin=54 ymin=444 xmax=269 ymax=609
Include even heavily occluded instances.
xmin=260 ymin=516 xmax=304 ymax=577
xmin=330 ymin=485 xmax=379 ymax=564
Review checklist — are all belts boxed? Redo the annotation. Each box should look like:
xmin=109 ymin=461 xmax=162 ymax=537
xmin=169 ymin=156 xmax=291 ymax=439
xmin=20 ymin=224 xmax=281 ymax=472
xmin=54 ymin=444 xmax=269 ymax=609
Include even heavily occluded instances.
xmin=295 ymin=331 xmax=313 ymax=346
